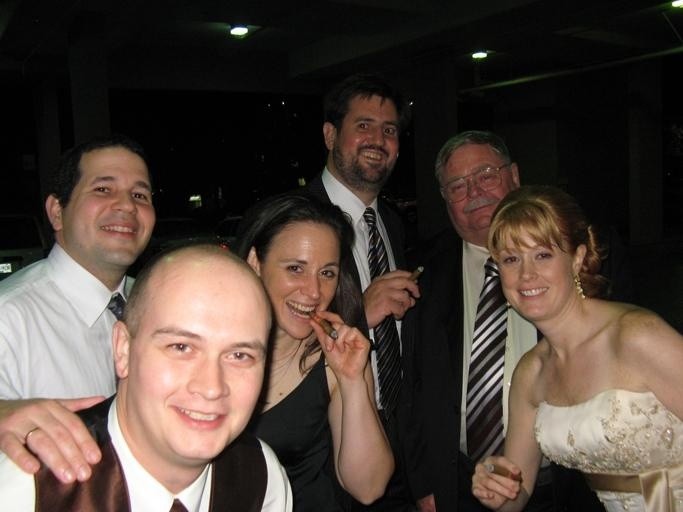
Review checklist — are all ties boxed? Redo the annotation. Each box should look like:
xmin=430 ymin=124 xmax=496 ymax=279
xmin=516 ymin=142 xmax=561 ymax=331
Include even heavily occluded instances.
xmin=107 ymin=293 xmax=127 ymax=322
xmin=168 ymin=498 xmax=189 ymax=512
xmin=362 ymin=205 xmax=405 ymax=429
xmin=463 ymin=252 xmax=510 ymax=468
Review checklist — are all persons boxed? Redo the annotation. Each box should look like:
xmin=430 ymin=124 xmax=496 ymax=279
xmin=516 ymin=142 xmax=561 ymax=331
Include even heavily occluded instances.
xmin=1 ymin=135 xmax=158 ymax=486
xmin=403 ymin=129 xmax=588 ymax=511
xmin=237 ymin=193 xmax=397 ymax=511
xmin=469 ymin=181 xmax=682 ymax=512
xmin=0 ymin=238 xmax=295 ymax=512
xmin=301 ymin=72 xmax=425 ymax=511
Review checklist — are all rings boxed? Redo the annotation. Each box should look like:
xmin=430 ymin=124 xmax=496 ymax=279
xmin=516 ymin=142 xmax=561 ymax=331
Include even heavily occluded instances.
xmin=24 ymin=425 xmax=40 ymax=446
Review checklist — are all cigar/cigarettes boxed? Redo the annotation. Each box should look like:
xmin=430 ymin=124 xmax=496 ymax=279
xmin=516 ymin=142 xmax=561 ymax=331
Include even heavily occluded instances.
xmin=405 ymin=264 xmax=426 ymax=280
xmin=307 ymin=308 xmax=340 ymax=340
xmin=482 ymin=459 xmax=526 ymax=484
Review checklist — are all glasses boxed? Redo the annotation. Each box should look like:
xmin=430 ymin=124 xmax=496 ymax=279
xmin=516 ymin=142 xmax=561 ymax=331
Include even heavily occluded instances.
xmin=438 ymin=161 xmax=512 ymax=204
